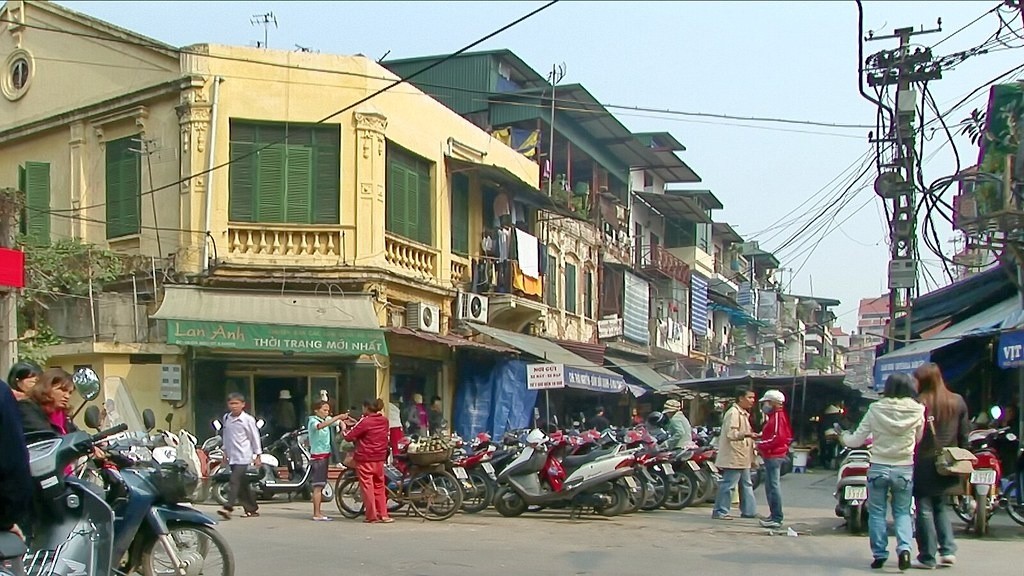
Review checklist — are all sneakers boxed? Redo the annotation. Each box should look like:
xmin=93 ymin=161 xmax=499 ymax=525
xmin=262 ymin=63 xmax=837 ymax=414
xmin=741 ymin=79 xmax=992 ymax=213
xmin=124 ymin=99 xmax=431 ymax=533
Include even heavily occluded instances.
xmin=918 ymin=555 xmax=936 ymax=569
xmin=942 ymin=555 xmax=955 ymax=564
xmin=759 ymin=517 xmax=783 ymax=528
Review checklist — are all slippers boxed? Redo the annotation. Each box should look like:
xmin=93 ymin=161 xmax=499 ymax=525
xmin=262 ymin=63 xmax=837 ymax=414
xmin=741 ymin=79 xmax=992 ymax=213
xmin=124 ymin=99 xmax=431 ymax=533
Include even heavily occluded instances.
xmin=240 ymin=512 xmax=259 ymax=518
xmin=217 ymin=510 xmax=231 ymax=520
xmin=313 ymin=512 xmax=333 ymax=521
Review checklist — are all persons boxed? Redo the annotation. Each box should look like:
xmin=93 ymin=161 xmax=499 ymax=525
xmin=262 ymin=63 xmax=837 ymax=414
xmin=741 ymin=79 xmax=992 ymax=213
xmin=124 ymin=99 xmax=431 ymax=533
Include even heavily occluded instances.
xmin=839 ymin=362 xmax=1017 ymax=571
xmin=273 ymin=388 xmax=296 ymax=467
xmin=585 ymin=406 xmax=609 ymax=432
xmin=659 ymin=399 xmax=695 ymax=448
xmin=319 ymin=389 xmax=348 ymax=469
xmin=0 ymin=359 xmax=107 ymax=541
xmin=712 ymin=385 xmax=794 ymax=527
xmin=428 ymin=395 xmax=448 ymax=436
xmin=388 ymin=393 xmax=430 ymax=477
xmin=308 ymin=397 xmax=396 ymax=523
xmin=217 ymin=392 xmax=262 ymax=520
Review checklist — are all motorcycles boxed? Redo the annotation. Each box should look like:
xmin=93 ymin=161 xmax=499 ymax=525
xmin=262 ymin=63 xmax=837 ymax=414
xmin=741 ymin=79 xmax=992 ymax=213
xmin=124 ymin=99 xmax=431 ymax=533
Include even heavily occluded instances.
xmin=834 ymin=405 xmax=1024 ymax=535
xmin=141 ymin=405 xmax=720 ymax=519
xmin=0 ymin=366 xmax=129 ymax=576
xmin=84 ymin=376 xmax=235 ymax=576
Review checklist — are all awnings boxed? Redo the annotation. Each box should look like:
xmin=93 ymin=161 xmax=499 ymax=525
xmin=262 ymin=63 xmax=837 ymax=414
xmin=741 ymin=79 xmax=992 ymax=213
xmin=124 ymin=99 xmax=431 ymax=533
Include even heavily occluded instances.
xmin=148 ymin=284 xmax=389 ymax=357
xmin=445 ymin=154 xmax=594 ymax=226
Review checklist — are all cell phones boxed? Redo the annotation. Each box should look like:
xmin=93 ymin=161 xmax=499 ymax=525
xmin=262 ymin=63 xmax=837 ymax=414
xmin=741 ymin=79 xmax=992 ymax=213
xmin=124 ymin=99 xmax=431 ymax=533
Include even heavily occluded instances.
xmin=834 ymin=423 xmax=843 ymax=433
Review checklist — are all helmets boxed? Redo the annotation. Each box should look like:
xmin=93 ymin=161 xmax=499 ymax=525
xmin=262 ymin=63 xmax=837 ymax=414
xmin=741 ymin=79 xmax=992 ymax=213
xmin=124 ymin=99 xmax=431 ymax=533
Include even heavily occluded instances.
xmin=649 ymin=412 xmax=665 ymax=425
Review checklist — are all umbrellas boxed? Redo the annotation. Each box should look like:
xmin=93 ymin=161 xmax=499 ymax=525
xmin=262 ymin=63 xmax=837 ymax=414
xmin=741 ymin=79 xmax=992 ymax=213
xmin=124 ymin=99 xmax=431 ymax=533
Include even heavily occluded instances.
xmin=872 ymin=293 xmax=1024 ymax=502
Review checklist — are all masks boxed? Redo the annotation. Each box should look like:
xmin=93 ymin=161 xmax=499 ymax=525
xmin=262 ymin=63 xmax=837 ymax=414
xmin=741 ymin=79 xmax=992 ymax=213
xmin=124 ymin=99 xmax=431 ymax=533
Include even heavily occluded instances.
xmin=762 ymin=404 xmax=772 ymax=413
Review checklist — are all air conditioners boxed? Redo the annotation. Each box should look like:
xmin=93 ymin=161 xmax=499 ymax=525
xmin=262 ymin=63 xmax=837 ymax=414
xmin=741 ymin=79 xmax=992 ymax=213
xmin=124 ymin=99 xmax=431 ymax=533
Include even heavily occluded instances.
xmin=455 ymin=293 xmax=488 ymax=325
xmin=407 ymin=302 xmax=439 ymax=334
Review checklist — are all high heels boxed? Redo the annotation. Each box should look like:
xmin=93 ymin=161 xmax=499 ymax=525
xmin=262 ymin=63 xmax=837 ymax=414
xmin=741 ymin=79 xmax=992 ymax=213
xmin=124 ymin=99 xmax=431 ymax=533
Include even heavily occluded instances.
xmin=899 ymin=550 xmax=910 ymax=570
xmin=871 ymin=557 xmax=887 ymax=569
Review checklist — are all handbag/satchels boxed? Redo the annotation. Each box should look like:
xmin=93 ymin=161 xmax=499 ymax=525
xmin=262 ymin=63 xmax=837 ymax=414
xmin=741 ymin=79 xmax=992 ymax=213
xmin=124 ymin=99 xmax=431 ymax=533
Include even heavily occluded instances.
xmin=935 ymin=448 xmax=978 ymax=476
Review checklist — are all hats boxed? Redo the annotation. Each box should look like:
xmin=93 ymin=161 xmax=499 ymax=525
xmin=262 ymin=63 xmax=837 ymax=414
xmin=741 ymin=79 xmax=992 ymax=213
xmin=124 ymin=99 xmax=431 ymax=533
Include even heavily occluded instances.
xmin=758 ymin=390 xmax=785 ymax=405
xmin=662 ymin=400 xmax=681 ymax=414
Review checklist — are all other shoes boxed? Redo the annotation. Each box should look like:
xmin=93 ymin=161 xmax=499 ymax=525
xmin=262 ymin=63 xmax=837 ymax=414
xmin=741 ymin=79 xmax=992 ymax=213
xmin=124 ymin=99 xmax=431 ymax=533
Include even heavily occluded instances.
xmin=363 ymin=519 xmax=395 ymax=524
xmin=740 ymin=513 xmax=765 ymax=518
xmin=712 ymin=517 xmax=733 ymax=520
